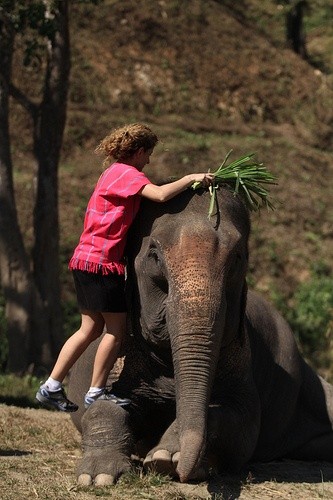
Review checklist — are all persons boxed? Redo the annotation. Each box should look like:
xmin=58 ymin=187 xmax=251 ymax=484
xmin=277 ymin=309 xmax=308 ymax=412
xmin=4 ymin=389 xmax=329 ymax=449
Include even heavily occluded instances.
xmin=34 ymin=121 xmax=218 ymax=414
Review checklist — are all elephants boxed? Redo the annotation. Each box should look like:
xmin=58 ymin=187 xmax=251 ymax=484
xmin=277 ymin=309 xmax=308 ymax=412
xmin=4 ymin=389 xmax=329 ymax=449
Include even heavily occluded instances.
xmin=67 ymin=171 xmax=332 ymax=499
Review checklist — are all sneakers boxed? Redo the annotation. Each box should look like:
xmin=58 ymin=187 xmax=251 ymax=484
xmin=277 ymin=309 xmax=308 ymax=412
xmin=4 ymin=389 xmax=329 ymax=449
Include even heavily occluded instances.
xmin=35 ymin=384 xmax=78 ymax=412
xmin=84 ymin=390 xmax=130 ymax=407
